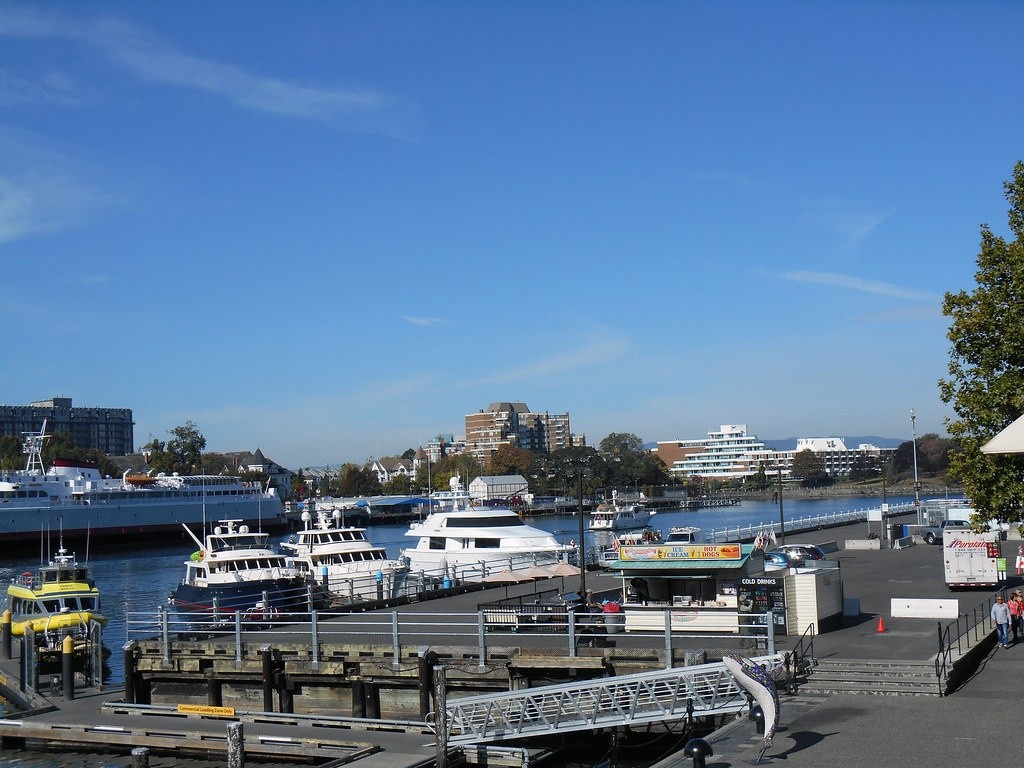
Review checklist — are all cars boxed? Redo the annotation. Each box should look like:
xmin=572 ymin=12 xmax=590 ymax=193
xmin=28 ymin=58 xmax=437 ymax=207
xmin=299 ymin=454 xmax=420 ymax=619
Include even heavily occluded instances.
xmin=768 ymin=548 xmax=814 ymax=568
xmin=764 ymin=551 xmax=791 ymax=570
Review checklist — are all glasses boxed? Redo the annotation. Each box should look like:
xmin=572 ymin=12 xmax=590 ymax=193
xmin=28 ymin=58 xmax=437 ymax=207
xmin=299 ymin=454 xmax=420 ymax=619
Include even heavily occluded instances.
xmin=1020 ymin=596 xmax=1022 ymax=597
xmin=590 ymin=591 xmax=593 ymax=594
xmin=997 ymin=599 xmax=1003 ymax=601
xmin=1015 ymin=596 xmax=1018 ymax=598
xmin=1019 ymin=592 xmax=1022 ymax=594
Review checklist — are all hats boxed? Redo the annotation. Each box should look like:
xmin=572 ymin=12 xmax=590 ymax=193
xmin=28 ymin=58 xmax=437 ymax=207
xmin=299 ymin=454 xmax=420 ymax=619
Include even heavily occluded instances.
xmin=586 ymin=589 xmax=592 ymax=593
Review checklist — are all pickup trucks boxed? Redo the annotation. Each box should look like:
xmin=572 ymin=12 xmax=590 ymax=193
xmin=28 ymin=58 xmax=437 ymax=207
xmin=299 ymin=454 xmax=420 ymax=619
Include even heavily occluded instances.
xmin=921 ymin=520 xmax=974 ymax=544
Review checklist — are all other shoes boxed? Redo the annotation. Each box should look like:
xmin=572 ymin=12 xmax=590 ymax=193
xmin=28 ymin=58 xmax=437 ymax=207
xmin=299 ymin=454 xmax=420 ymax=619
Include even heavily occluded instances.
xmin=999 ymin=642 xmax=1004 ymax=648
xmin=1003 ymin=645 xmax=1008 ymax=649
xmin=739 ymin=644 xmax=749 ymax=649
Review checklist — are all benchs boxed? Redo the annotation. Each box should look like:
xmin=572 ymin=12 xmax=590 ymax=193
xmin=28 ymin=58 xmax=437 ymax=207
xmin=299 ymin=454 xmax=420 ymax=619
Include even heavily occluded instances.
xmin=482 ymin=598 xmax=604 ymax=633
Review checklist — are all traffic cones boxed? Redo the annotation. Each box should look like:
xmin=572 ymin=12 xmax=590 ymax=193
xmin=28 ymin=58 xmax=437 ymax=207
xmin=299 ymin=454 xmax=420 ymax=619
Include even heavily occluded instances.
xmin=876 ymin=617 xmax=885 ymax=632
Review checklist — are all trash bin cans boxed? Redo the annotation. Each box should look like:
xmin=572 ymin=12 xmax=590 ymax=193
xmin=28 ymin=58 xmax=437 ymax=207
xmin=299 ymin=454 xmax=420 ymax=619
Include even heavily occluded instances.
xmin=602 ymin=603 xmax=622 ymax=635
xmin=903 ymin=523 xmax=913 ymax=537
xmin=894 ymin=524 xmax=903 ymax=539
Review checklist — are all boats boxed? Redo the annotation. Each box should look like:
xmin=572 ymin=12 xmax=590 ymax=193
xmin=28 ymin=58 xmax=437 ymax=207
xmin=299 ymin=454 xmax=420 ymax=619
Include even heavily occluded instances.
xmin=166 ymin=518 xmax=331 ymax=629
xmin=586 ymin=491 xmax=657 ymax=530
xmin=0 ymin=514 xmax=113 ymax=673
xmin=126 ymin=476 xmax=158 ymax=485
xmin=397 ymin=477 xmax=579 ymax=583
xmin=278 ymin=506 xmax=415 ymax=606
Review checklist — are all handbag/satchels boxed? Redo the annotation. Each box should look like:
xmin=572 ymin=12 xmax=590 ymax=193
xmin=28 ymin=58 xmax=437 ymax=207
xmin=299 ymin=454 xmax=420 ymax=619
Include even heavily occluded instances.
xmin=588 ymin=641 xmax=594 ymax=648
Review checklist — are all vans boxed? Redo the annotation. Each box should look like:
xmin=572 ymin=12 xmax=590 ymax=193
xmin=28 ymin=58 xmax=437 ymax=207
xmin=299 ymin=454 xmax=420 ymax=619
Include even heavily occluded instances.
xmin=662 ymin=527 xmax=707 ymax=547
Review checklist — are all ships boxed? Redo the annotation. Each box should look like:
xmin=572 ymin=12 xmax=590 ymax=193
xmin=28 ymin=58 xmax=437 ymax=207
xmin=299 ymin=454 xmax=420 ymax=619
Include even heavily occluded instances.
xmin=0 ymin=416 xmax=291 ymax=539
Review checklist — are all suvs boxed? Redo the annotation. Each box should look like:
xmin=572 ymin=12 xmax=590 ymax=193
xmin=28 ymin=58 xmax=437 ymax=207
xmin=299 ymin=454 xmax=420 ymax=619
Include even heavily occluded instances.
xmin=778 ymin=543 xmax=826 ymax=561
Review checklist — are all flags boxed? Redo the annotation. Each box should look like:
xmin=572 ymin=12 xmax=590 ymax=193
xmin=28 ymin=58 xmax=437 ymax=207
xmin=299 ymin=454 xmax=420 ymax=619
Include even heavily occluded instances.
xmin=753 ymin=528 xmax=777 ymax=550
xmin=611 ymin=534 xmax=635 ymax=552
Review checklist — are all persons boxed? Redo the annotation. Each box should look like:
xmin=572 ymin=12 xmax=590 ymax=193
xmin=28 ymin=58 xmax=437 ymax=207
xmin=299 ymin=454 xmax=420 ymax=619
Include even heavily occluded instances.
xmin=618 ymin=591 xmax=623 ymax=603
xmin=991 ymin=594 xmax=1012 ymax=649
xmin=586 ymin=589 xmax=603 ymax=620
xmin=1007 ymin=590 xmax=1024 ymax=643
xmin=737 ymin=595 xmax=754 ymax=649
xmin=578 ymin=621 xmax=609 ymax=647
